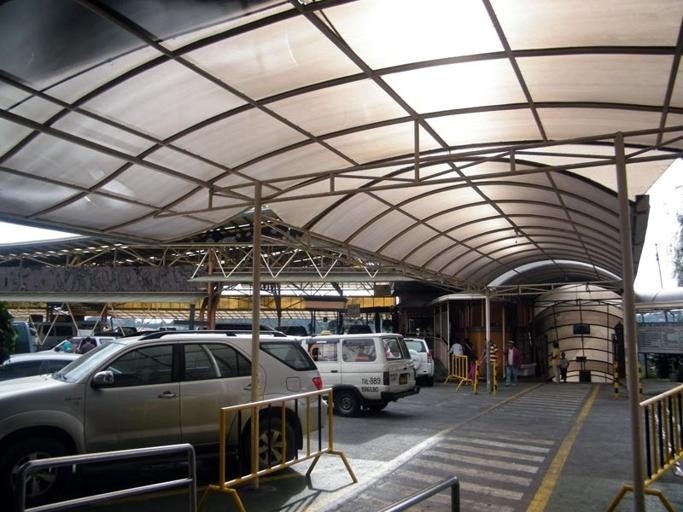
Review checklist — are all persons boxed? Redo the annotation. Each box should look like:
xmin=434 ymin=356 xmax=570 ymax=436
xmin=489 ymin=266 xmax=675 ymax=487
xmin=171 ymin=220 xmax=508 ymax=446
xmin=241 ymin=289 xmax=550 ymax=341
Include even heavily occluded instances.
xmin=547 ymin=342 xmax=560 ymax=383
xmin=501 ymin=340 xmax=523 ymax=387
xmin=80 ymin=337 xmax=95 ymax=353
xmin=447 ymin=337 xmax=462 ymax=355
xmin=559 ymin=351 xmax=569 ymax=382
xmin=478 ymin=337 xmax=498 ymax=366
xmin=467 ymin=361 xmax=478 ymax=389
xmin=63 ymin=336 xmax=78 ymax=353
xmin=462 ymin=335 xmax=475 ymax=365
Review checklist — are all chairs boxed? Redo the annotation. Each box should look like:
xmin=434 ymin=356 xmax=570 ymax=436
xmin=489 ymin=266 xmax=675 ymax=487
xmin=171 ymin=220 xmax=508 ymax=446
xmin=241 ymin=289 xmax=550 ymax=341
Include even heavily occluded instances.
xmin=311 ymin=348 xmax=322 ymax=361
xmin=127 ymin=349 xmax=160 ymax=383
